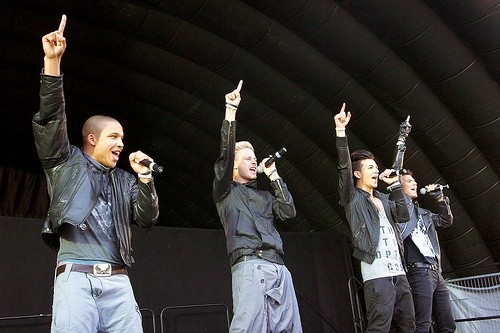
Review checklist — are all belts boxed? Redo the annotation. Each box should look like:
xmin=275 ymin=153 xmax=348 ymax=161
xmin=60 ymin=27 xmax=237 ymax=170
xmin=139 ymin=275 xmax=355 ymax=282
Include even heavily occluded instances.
xmin=56 ymin=263 xmax=129 ymax=277
xmin=235 ymin=255 xmax=262 ymax=266
xmin=407 ymin=262 xmax=439 ymax=270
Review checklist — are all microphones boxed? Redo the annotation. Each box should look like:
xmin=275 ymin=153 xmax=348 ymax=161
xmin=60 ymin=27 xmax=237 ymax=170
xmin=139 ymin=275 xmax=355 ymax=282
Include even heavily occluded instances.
xmin=256 ymin=147 xmax=287 ymax=172
xmin=420 ymin=184 xmax=448 ymax=194
xmin=129 ymin=153 xmax=163 ymax=173
xmin=379 ymin=168 xmax=406 ymax=180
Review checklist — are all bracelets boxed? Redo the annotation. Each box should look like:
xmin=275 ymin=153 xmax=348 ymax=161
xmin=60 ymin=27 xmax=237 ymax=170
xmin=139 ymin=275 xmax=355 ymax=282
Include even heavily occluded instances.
xmin=398 ymin=139 xmax=405 ymax=143
xmin=267 ymin=169 xmax=276 ymax=177
xmin=225 ymin=104 xmax=237 ymax=110
xmin=226 ymin=102 xmax=238 ymax=108
xmin=386 ymin=182 xmax=401 ymax=190
xmin=142 ymin=172 xmax=150 ymax=175
xmin=137 ymin=174 xmax=151 ymax=178
xmin=335 ymin=128 xmax=346 ymax=130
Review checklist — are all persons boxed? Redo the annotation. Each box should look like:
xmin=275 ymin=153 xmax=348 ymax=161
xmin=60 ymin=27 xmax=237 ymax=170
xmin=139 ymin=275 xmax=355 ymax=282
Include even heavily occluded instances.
xmin=392 ymin=115 xmax=457 ymax=333
xmin=213 ymin=80 xmax=303 ymax=333
xmin=334 ymin=102 xmax=416 ymax=333
xmin=33 ymin=15 xmax=158 ymax=333
